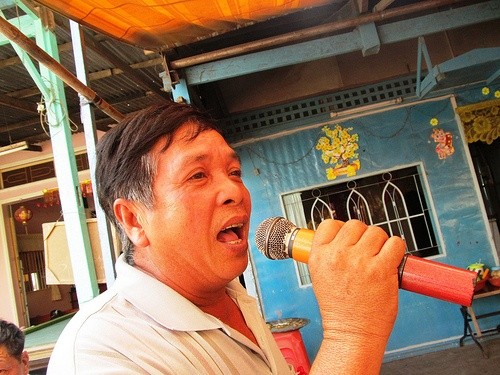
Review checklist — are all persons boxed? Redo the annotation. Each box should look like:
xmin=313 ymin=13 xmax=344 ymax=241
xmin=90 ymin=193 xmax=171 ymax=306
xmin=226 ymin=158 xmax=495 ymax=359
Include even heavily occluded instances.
xmin=47 ymin=102 xmax=407 ymax=375
xmin=0 ymin=319 xmax=30 ymax=375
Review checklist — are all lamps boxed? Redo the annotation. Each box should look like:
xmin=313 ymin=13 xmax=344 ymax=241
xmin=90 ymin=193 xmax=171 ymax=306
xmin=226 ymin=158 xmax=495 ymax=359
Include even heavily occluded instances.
xmin=0 ymin=141 xmax=29 ymax=156
xmin=330 ymin=97 xmax=402 ymax=118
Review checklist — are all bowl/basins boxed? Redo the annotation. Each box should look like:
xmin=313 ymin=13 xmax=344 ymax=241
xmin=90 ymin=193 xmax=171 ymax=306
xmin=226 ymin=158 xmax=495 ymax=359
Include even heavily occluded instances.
xmin=474 ymin=277 xmax=488 ymax=292
xmin=488 ymin=273 xmax=500 ymax=286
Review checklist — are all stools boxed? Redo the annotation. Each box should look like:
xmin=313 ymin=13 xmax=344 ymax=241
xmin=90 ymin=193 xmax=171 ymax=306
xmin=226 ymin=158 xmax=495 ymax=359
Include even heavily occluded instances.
xmin=273 ymin=329 xmax=312 ymax=375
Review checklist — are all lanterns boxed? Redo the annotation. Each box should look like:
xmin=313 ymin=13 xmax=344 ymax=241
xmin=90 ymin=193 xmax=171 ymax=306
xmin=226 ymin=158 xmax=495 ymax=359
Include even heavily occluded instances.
xmin=15 ymin=206 xmax=32 ymax=237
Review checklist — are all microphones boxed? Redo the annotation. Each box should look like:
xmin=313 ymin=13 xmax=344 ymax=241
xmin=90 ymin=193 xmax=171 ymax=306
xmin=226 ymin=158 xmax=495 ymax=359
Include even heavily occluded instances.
xmin=255 ymin=216 xmax=478 ymax=306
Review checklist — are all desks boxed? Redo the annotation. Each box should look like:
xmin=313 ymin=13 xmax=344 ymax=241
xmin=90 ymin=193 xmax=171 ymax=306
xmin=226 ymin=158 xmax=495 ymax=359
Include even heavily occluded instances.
xmin=460 ymin=285 xmax=500 ymax=359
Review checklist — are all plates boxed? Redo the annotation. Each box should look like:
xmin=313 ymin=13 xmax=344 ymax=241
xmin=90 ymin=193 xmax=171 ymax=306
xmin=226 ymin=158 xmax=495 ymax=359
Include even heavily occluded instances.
xmin=265 ymin=318 xmax=311 ymax=332
